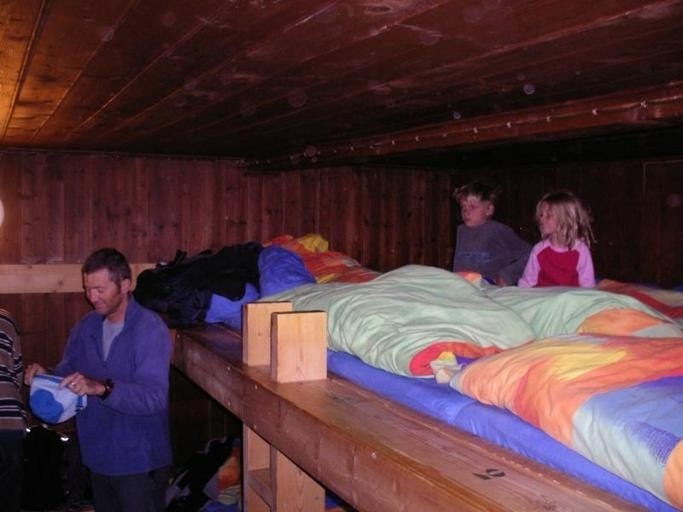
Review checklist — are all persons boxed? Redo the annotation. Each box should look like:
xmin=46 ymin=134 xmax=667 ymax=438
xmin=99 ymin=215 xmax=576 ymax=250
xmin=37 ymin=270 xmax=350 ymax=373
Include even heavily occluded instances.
xmin=24 ymin=247 xmax=171 ymax=512
xmin=516 ymin=189 xmax=600 ymax=288
xmin=448 ymin=181 xmax=533 ymax=287
xmin=0 ymin=308 xmax=30 ymax=512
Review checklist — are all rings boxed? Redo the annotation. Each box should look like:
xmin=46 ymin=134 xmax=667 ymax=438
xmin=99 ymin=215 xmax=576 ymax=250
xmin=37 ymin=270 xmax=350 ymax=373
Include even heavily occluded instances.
xmin=70 ymin=380 xmax=75 ymax=387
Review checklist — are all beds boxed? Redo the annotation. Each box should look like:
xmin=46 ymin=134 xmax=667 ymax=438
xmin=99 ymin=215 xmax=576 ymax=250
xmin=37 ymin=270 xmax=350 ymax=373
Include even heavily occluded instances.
xmin=169 ymin=233 xmax=683 ymax=512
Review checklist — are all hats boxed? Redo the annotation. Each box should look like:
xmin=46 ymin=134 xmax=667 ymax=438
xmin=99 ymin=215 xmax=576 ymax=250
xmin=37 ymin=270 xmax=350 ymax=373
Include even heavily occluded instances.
xmin=28 ymin=372 xmax=89 ymax=426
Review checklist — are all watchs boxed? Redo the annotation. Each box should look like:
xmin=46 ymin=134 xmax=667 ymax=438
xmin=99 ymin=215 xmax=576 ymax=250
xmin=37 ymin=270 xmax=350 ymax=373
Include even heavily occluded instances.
xmin=97 ymin=377 xmax=114 ymax=402
